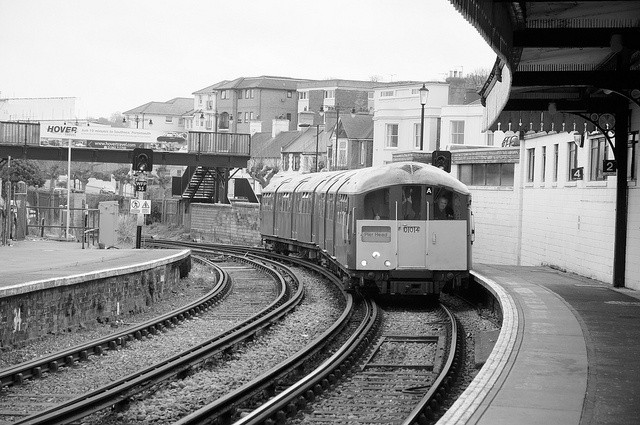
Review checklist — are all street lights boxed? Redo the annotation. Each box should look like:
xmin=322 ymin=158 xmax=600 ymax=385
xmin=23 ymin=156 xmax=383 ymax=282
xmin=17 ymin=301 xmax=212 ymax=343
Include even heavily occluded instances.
xmin=419 ymin=84 xmax=429 ymax=151
xmin=320 ymin=103 xmax=355 ymax=171
xmin=123 ymin=116 xmax=153 ymax=129
xmin=299 ymin=124 xmax=320 ymax=172
xmin=201 ymin=111 xmax=233 ymax=133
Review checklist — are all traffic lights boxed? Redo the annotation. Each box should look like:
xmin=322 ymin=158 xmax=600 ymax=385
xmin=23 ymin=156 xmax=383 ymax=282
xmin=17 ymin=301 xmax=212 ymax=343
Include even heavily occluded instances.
xmin=132 ymin=148 xmax=153 ymax=171
xmin=431 ymin=151 xmax=451 ymax=173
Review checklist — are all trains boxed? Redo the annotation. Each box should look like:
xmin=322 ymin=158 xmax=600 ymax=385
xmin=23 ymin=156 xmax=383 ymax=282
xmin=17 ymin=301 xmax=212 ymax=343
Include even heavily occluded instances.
xmin=259 ymin=161 xmax=475 ymax=302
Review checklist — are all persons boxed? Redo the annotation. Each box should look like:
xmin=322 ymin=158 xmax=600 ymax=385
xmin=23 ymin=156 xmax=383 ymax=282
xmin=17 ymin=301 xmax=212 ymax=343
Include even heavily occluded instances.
xmin=436 ymin=196 xmax=455 ymax=220
